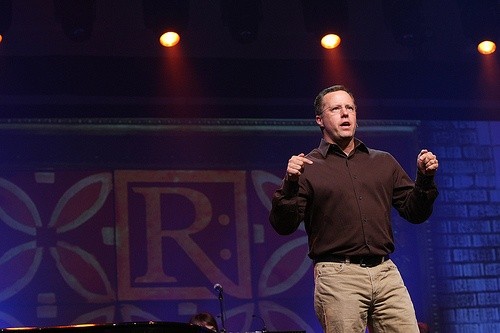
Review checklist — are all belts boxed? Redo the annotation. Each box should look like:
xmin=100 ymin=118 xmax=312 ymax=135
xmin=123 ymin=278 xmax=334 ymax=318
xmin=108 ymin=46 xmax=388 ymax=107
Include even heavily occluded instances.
xmin=312 ymin=252 xmax=390 ymax=268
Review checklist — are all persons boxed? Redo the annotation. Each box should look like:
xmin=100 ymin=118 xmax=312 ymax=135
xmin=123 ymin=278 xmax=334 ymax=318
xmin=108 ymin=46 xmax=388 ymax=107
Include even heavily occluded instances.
xmin=268 ymin=85 xmax=439 ymax=333
xmin=191 ymin=311 xmax=219 ymax=333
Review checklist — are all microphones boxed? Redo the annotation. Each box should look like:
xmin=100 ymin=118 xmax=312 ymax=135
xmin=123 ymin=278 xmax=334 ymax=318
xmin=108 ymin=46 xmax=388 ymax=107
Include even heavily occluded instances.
xmin=214 ymin=284 xmax=223 ymax=299
xmin=356 ymin=124 xmax=358 ymax=128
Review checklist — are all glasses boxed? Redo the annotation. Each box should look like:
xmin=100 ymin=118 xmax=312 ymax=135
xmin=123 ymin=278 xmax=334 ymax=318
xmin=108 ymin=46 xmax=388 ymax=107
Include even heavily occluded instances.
xmin=318 ymin=104 xmax=357 ymax=116
